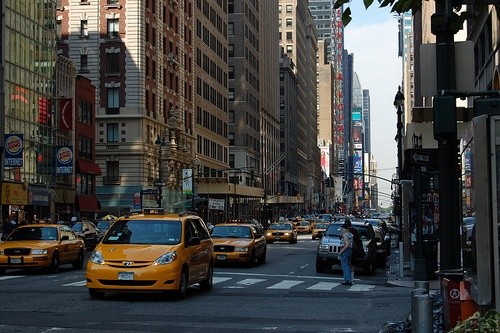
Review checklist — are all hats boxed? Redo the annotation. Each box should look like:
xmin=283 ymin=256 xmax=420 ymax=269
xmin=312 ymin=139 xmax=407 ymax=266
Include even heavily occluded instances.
xmin=341 ymin=224 xmax=350 ymax=229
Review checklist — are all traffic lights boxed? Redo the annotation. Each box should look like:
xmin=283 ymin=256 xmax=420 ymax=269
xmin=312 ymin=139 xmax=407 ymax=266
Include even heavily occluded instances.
xmin=325 ymin=177 xmax=334 ymax=187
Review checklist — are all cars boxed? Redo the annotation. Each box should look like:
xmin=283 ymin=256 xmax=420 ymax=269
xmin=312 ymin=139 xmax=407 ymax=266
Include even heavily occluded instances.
xmin=60 ymin=221 xmax=100 ymax=254
xmin=0 ymin=225 xmax=86 ymax=271
xmin=91 ymin=219 xmax=115 ymax=240
xmin=296 ymin=213 xmax=357 ymax=223
xmin=85 ymin=207 xmax=214 ymax=299
xmin=209 ymin=222 xmax=267 ymax=265
xmin=312 ymin=224 xmax=328 ymax=239
xmin=363 ymin=212 xmax=399 ymax=263
xmin=463 ymin=217 xmax=475 ymax=233
xmin=266 ymin=223 xmax=297 ymax=244
xmin=295 ymin=221 xmax=312 ymax=233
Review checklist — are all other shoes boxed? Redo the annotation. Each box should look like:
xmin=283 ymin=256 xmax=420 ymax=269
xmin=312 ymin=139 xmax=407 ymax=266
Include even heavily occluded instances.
xmin=342 ymin=282 xmax=353 ymax=286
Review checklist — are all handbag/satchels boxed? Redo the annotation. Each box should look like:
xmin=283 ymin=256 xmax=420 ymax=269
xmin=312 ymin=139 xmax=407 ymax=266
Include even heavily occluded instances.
xmin=356 ymin=245 xmax=366 ymax=257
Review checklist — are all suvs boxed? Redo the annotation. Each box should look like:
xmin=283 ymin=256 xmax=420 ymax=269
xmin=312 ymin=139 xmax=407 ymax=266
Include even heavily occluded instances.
xmin=315 ymin=220 xmax=380 ymax=275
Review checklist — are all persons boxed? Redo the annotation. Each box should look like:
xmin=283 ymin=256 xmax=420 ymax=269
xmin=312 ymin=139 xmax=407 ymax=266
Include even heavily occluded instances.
xmin=208 ymin=223 xmax=214 ymax=232
xmin=338 ymin=219 xmax=361 ymax=285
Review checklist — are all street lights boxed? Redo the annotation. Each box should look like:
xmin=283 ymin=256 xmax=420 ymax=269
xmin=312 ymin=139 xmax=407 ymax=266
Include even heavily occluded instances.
xmin=155 ymin=134 xmax=167 ymax=208
xmin=394 ymin=86 xmax=405 ymax=180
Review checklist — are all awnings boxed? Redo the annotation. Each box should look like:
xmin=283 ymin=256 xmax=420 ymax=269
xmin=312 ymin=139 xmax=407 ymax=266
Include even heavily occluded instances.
xmin=77 ymin=195 xmax=99 ymax=212
xmin=78 ymin=160 xmax=102 ymax=176
